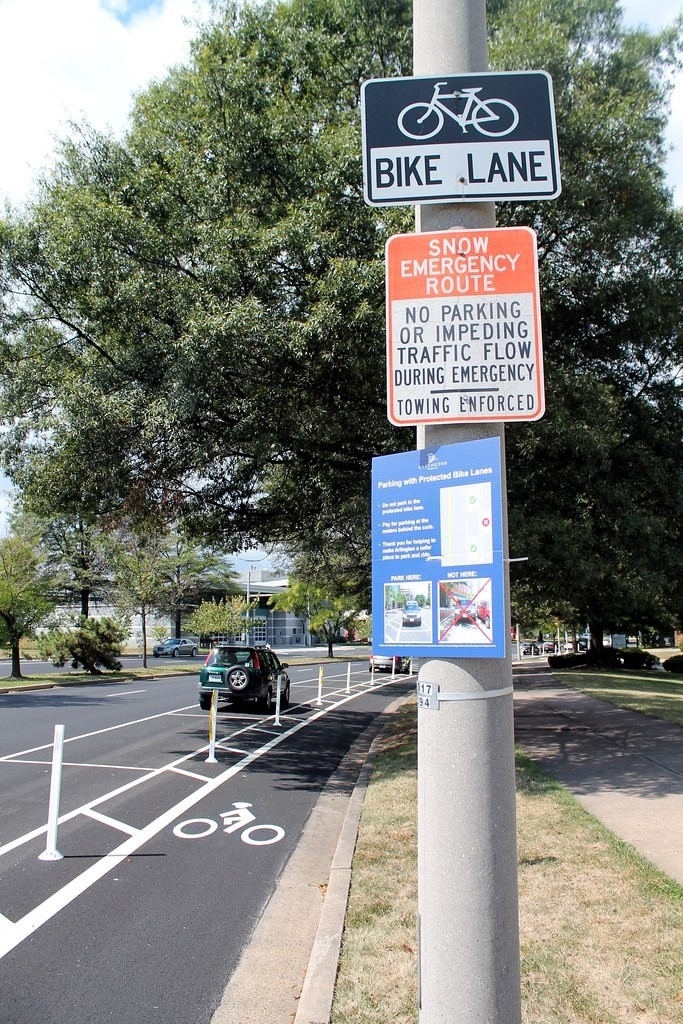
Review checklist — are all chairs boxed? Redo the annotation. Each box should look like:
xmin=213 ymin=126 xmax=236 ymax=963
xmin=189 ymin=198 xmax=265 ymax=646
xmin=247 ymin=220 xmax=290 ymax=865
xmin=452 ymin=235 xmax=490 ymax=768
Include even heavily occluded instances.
xmin=227 ymin=654 xmax=238 ymax=664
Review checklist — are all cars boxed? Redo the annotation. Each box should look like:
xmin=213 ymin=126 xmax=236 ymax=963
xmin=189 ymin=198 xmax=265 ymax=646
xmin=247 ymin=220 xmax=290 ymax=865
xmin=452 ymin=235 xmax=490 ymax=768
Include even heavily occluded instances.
xmin=564 ymin=640 xmax=583 ymax=651
xmin=579 ymin=633 xmax=589 ymax=647
xmin=153 ymin=638 xmax=198 ymax=657
xmin=453 ymin=599 xmax=476 ymax=622
xmin=401 ymin=603 xmax=421 ymax=626
xmin=520 ymin=643 xmax=539 ymax=655
xmin=541 ymin=642 xmax=561 ymax=653
xmin=369 ymin=653 xmax=409 ymax=673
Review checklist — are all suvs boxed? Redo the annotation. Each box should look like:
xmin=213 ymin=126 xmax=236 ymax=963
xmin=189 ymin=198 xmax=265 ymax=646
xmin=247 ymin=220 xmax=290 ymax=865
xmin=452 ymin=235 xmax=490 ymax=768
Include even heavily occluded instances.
xmin=197 ymin=644 xmax=290 ymax=710
